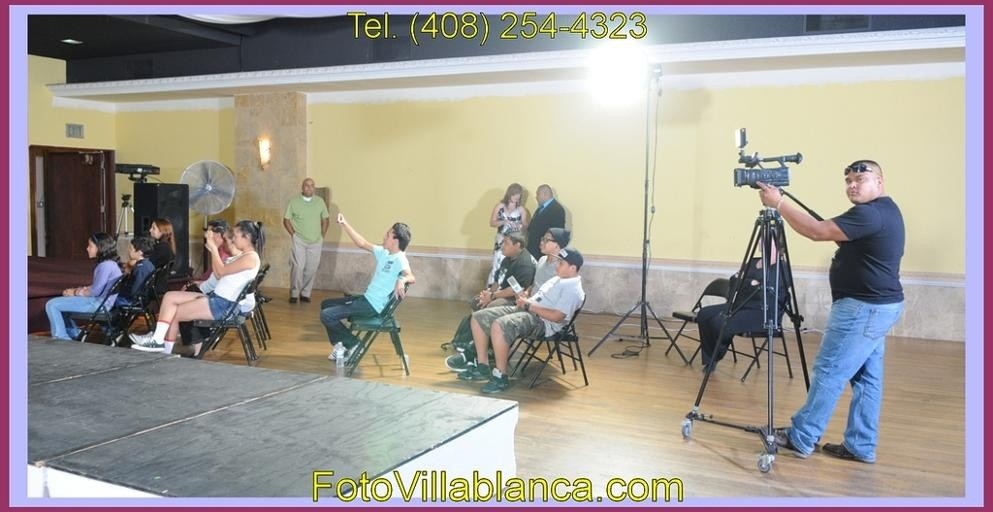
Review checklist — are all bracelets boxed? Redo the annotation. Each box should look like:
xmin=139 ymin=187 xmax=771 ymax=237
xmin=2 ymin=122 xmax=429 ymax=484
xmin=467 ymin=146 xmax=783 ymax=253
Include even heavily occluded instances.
xmin=776 ymin=196 xmax=787 ymax=216
xmin=73 ymin=289 xmax=79 ymax=296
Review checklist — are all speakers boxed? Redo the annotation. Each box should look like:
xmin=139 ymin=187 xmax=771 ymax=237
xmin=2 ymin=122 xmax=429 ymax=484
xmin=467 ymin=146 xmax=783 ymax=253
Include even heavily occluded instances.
xmin=132 ymin=184 xmax=191 ymax=266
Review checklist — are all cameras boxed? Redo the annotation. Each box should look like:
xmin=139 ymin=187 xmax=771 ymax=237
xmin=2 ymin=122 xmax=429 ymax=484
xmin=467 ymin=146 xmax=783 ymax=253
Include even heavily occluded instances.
xmin=734 ymin=128 xmax=803 ymax=189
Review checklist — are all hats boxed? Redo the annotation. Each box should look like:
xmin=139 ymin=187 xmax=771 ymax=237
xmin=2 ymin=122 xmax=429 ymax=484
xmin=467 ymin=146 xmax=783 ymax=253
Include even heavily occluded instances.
xmin=551 ymin=248 xmax=583 ymax=269
xmin=549 ymin=227 xmax=571 ymax=248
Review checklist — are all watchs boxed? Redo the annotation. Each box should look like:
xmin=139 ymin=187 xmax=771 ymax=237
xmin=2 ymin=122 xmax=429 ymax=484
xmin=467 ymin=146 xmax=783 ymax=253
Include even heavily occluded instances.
xmin=490 ymin=292 xmax=496 ymax=300
xmin=524 ymin=302 xmax=531 ymax=311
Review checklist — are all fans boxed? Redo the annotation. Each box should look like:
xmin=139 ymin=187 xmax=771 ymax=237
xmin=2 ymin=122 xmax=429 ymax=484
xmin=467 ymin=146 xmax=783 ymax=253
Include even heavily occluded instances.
xmin=179 ymin=159 xmax=235 ymax=274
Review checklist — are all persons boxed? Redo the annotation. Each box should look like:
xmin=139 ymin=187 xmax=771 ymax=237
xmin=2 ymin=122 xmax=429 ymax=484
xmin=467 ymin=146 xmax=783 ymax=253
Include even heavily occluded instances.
xmin=179 ymin=219 xmax=232 ymax=360
xmin=446 ymin=227 xmax=572 ymax=372
xmin=147 ymin=218 xmax=177 ymax=268
xmin=99 ymin=235 xmax=156 ymax=346
xmin=486 ymin=182 xmax=528 ymax=290
xmin=529 ymin=184 xmax=569 ymax=261
xmin=456 ymin=231 xmax=539 ymax=358
xmin=319 ymin=213 xmax=416 ymax=365
xmin=44 ymin=231 xmax=125 ymax=344
xmin=128 ymin=219 xmax=262 ymax=356
xmin=756 ymin=160 xmax=906 ymax=464
xmin=283 ymin=178 xmax=330 ymax=303
xmin=456 ymin=248 xmax=585 ymax=395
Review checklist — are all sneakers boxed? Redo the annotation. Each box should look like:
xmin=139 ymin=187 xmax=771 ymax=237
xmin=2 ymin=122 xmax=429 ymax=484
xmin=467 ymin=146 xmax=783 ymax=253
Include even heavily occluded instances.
xmin=129 ymin=333 xmax=154 ymax=345
xmin=458 ymin=361 xmax=492 ymax=381
xmin=131 ymin=339 xmax=166 ymax=352
xmin=329 ymin=346 xmax=346 ymax=360
xmin=336 ymin=344 xmax=366 ymax=363
xmin=481 ymin=369 xmax=509 ymax=394
xmin=442 ymin=337 xmax=475 ymax=369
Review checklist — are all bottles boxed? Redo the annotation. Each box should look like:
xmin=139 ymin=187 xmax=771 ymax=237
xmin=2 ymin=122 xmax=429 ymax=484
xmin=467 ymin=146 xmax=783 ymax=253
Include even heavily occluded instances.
xmin=336 ymin=342 xmax=343 ymax=368
xmin=507 ymin=276 xmax=526 ymax=300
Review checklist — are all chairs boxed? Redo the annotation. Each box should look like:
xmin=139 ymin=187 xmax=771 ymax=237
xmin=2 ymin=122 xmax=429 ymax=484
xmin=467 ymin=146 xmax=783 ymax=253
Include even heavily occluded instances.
xmin=161 ymin=259 xmax=177 ymax=292
xmin=510 ymin=291 xmax=587 ymax=389
xmin=345 ymin=280 xmax=411 ymax=375
xmin=196 ymin=280 xmax=257 ymax=365
xmin=219 ymin=263 xmax=272 ymax=341
xmin=111 ymin=272 xmax=161 ymax=347
xmin=209 ymin=272 xmax=266 ymax=351
xmin=75 ymin=273 xmax=131 ymax=347
xmin=729 ymin=291 xmax=794 ymax=382
xmin=666 ymin=278 xmax=739 ymax=365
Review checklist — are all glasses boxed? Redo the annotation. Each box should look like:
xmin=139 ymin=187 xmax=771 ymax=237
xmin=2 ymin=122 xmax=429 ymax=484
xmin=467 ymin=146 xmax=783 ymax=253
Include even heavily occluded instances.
xmin=541 ymin=235 xmax=557 ymax=244
xmin=845 ymin=163 xmax=877 ymax=176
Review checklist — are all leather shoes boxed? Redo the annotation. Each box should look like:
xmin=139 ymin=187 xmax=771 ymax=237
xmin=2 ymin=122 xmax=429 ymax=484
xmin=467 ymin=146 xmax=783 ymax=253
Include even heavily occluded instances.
xmin=289 ymin=296 xmax=298 ymax=302
xmin=767 ymin=431 xmax=806 ymax=459
xmin=301 ymin=296 xmax=310 ymax=301
xmin=825 ymin=443 xmax=863 ymax=460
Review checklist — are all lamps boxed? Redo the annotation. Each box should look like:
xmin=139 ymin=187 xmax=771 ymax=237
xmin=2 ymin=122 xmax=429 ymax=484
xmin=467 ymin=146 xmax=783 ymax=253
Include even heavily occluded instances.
xmin=258 ymin=138 xmax=272 ymax=168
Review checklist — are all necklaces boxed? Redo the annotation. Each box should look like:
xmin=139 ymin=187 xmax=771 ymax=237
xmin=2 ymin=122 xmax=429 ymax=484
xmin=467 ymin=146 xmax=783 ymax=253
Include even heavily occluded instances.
xmin=243 ymin=249 xmax=254 ymax=253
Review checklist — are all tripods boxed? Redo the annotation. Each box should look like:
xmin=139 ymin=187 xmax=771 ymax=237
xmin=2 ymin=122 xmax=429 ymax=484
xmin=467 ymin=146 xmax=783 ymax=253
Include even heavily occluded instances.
xmin=114 ymin=196 xmax=135 ymax=242
xmin=588 ymin=65 xmax=691 ymax=365
xmin=684 ymin=187 xmax=810 ymax=473
